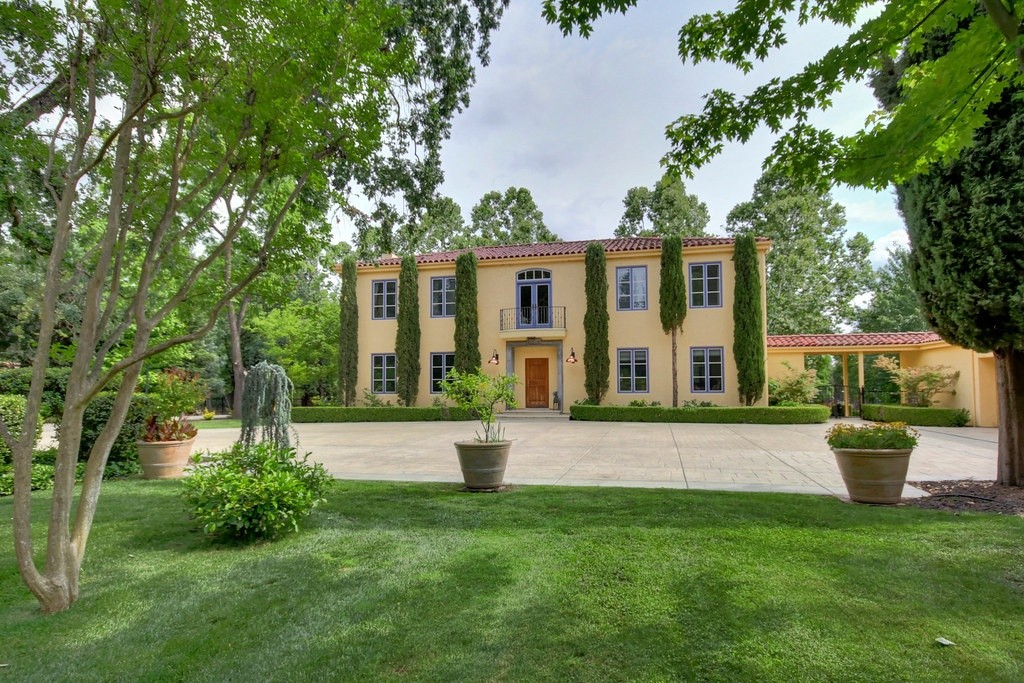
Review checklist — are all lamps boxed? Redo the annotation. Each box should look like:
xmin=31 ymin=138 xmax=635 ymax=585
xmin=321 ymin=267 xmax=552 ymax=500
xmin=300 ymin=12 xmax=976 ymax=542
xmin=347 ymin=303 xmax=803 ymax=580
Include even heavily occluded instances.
xmin=566 ymin=347 xmax=578 ymax=363
xmin=488 ymin=349 xmax=499 ymax=366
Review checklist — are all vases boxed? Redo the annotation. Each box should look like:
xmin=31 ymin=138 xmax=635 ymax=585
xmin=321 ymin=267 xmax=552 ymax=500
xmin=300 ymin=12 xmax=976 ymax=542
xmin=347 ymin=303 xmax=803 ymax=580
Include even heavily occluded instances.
xmin=833 ymin=448 xmax=913 ymax=504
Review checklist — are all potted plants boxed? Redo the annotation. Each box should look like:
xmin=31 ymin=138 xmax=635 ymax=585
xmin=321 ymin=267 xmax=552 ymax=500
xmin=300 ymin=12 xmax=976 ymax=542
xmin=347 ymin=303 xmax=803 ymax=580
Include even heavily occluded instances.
xmin=135 ymin=366 xmax=212 ymax=480
xmin=436 ymin=367 xmax=524 ymax=488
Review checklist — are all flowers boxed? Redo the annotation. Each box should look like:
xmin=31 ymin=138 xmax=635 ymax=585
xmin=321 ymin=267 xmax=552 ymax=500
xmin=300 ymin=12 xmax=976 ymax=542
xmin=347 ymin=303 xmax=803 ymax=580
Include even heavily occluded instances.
xmin=824 ymin=421 xmax=922 ymax=449
